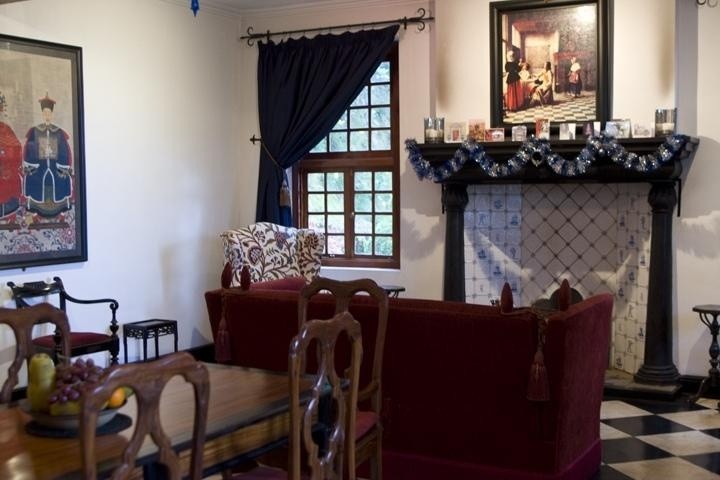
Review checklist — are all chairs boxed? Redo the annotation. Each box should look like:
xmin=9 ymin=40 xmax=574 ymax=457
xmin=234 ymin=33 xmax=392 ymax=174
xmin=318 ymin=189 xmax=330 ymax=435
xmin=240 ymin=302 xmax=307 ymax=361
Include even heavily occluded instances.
xmin=215 ymin=221 xmax=323 ymax=293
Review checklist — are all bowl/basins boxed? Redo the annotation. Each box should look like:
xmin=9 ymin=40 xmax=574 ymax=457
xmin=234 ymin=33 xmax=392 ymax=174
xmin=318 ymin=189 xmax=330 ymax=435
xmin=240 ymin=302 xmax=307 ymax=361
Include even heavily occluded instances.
xmin=16 ymin=399 xmax=130 ymax=431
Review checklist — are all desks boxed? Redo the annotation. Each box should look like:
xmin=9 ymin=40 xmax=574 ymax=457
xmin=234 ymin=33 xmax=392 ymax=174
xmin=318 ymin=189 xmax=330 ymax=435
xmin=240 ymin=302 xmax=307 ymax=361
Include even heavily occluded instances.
xmin=685 ymin=303 xmax=720 ymax=411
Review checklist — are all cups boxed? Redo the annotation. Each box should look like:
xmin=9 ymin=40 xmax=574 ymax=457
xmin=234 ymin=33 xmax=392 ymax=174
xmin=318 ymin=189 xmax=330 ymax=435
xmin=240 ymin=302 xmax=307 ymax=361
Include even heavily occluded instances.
xmin=422 ymin=115 xmax=446 ymax=145
xmin=655 ymin=108 xmax=678 ymax=136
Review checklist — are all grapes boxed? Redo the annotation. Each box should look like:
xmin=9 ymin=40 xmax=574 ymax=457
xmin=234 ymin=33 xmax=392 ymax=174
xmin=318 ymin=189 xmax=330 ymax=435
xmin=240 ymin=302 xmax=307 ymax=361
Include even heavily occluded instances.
xmin=48 ymin=358 xmax=105 ymax=405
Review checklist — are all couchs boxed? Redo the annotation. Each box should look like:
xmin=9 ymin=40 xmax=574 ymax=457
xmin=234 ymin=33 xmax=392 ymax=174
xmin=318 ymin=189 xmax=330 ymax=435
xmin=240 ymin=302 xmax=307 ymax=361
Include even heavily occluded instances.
xmin=204 ymin=273 xmax=612 ymax=479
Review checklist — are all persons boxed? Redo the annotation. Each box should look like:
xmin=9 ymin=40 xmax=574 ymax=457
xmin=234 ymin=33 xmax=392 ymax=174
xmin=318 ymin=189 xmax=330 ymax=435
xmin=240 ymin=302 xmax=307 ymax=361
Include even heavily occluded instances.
xmin=530 ymin=62 xmax=553 ymax=106
xmin=506 ymin=51 xmax=524 ymax=111
xmin=0 ymin=93 xmax=23 ymax=220
xmin=567 ymin=57 xmax=581 ymax=97
xmin=519 ymin=63 xmax=529 ymax=80
xmin=25 ymin=91 xmax=72 ymax=217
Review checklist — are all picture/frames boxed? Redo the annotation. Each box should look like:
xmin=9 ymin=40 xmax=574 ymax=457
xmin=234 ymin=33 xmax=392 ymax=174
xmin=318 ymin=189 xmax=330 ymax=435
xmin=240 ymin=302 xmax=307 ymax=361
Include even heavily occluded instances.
xmin=490 ymin=0 xmax=614 ymax=130
xmin=0 ymin=33 xmax=88 ymax=268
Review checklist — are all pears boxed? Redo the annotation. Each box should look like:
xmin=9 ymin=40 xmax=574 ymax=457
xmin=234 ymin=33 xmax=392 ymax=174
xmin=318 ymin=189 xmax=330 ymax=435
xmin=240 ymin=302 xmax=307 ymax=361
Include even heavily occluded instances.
xmin=51 ymin=400 xmax=82 ymax=417
xmin=28 ymin=351 xmax=56 ymax=414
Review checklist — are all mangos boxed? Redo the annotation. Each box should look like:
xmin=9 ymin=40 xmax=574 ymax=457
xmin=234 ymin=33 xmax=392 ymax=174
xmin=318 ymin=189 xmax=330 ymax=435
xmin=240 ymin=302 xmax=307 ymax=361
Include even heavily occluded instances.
xmin=109 ymin=389 xmax=125 ymax=408
